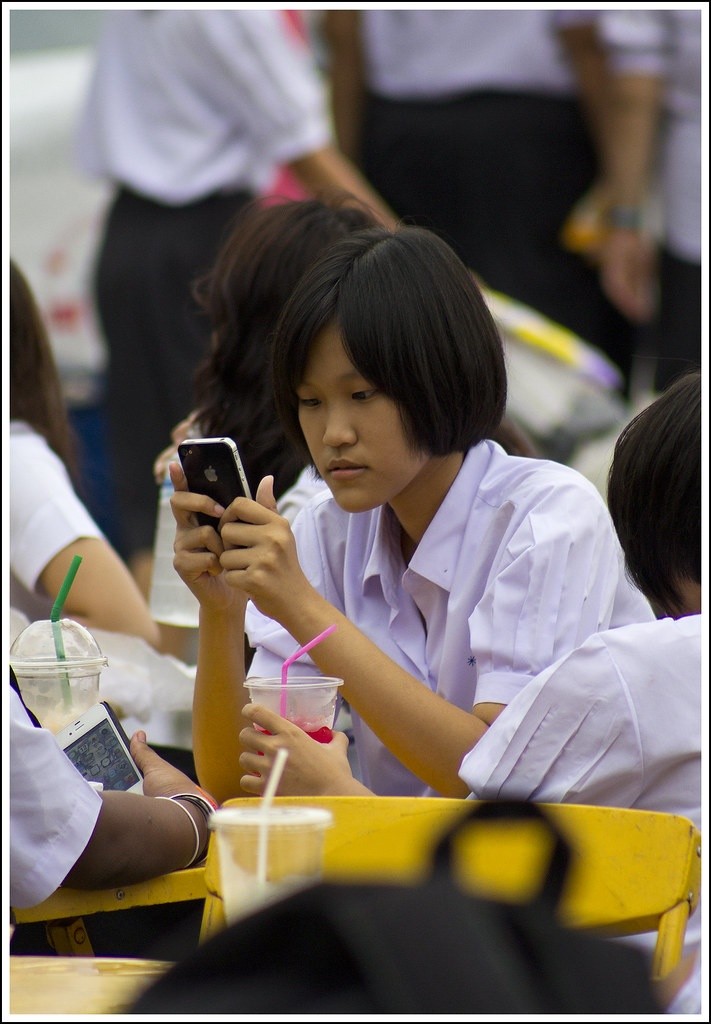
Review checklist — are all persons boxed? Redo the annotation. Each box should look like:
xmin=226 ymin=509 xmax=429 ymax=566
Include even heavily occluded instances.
xmin=456 ymin=373 xmax=701 ymax=1010
xmin=9 ymin=664 xmax=219 ymax=906
xmin=168 ymin=227 xmax=652 ymax=803
xmin=84 ymin=10 xmax=699 ymax=505
xmin=9 ymin=252 xmax=162 ymax=646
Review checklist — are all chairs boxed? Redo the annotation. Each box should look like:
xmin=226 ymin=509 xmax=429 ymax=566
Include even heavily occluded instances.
xmin=2 ymin=796 xmax=701 ymax=1010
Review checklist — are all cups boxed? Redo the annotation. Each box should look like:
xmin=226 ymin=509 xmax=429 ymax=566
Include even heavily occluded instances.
xmin=206 ymin=806 xmax=331 ymax=923
xmin=242 ymin=676 xmax=344 ymax=796
xmin=10 ymin=618 xmax=108 ymax=735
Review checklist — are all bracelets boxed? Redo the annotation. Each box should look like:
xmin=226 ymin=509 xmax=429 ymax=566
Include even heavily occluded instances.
xmin=156 ymin=791 xmax=219 ymax=870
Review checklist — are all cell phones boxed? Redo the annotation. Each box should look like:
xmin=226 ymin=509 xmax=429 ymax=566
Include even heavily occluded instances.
xmin=177 ymin=438 xmax=254 ymax=554
xmin=55 ymin=701 xmax=145 ymax=796
